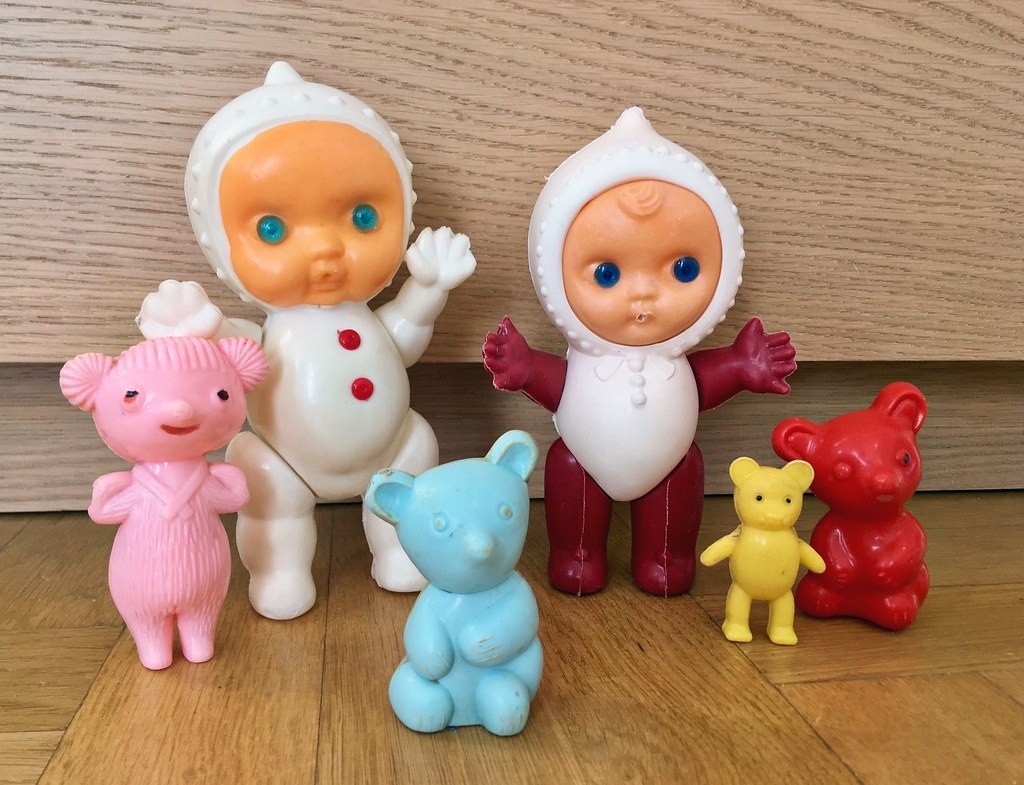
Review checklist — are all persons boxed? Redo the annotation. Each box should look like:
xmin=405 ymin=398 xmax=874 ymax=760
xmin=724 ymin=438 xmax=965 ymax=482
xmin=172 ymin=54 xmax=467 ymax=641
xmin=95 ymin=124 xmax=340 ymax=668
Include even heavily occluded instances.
xmin=483 ymin=106 xmax=798 ymax=599
xmin=134 ymin=61 xmax=480 ymax=621
